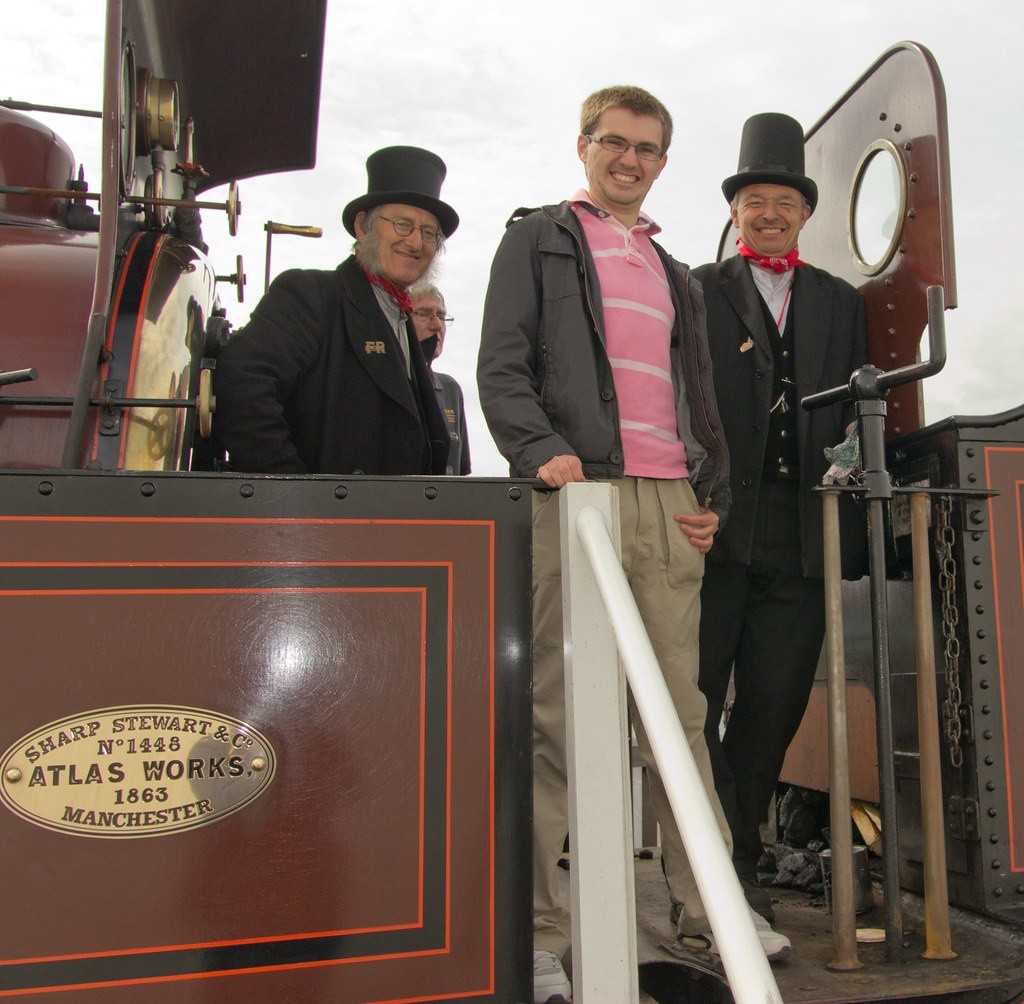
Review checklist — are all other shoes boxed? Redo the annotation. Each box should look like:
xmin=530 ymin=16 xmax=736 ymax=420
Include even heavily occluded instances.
xmin=737 ymin=871 xmax=776 ymax=919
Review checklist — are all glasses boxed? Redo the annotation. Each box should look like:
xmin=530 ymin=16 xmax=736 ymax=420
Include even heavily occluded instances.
xmin=413 ymin=310 xmax=455 ymax=328
xmin=585 ymin=133 xmax=663 ymax=161
xmin=374 ymin=212 xmax=441 ymax=243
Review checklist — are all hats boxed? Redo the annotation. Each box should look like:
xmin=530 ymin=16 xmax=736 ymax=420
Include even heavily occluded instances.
xmin=342 ymin=146 xmax=460 ymax=240
xmin=722 ymin=112 xmax=817 ymax=218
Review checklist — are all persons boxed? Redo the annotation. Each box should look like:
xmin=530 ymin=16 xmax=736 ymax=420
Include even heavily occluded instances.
xmin=198 ymin=142 xmax=472 ymax=476
xmin=472 ymin=86 xmax=801 ymax=1003
xmin=668 ymin=110 xmax=872 ymax=924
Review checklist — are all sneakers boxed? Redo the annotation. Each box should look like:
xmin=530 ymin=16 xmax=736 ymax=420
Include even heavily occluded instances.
xmin=680 ymin=924 xmax=792 ymax=963
xmin=532 ymin=947 xmax=571 ymax=1004
